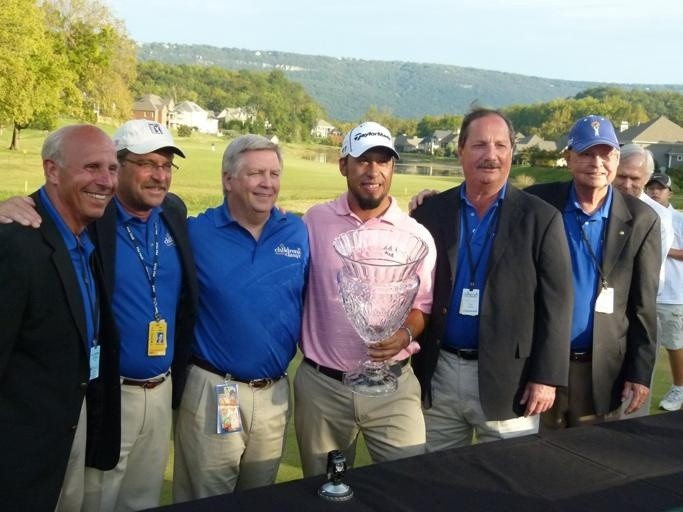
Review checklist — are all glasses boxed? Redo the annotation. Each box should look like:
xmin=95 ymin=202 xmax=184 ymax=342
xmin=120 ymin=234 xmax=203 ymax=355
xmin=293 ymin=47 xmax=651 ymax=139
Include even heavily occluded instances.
xmin=122 ymin=158 xmax=179 ymax=173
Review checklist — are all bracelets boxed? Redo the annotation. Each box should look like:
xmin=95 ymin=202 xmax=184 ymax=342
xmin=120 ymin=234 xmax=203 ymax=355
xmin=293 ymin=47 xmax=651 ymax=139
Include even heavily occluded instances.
xmin=398 ymin=323 xmax=416 ymax=347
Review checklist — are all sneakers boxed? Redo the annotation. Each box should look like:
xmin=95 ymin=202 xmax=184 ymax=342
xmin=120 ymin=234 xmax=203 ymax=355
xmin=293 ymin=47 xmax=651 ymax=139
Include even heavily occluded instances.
xmin=657 ymin=386 xmax=683 ymax=412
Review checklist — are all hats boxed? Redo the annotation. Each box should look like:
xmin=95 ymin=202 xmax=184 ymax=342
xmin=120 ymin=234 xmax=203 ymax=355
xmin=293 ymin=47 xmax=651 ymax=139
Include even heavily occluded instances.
xmin=645 ymin=173 xmax=671 ymax=191
xmin=568 ymin=115 xmax=620 ymax=153
xmin=340 ymin=122 xmax=401 ymax=160
xmin=111 ymin=119 xmax=191 ymax=160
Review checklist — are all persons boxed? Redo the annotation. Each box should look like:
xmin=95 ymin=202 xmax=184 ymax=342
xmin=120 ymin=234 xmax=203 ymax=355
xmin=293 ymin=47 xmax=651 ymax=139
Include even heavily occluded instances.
xmin=644 ymin=172 xmax=683 ymax=411
xmin=407 ymin=115 xmax=662 ymax=429
xmin=0 ymin=122 xmax=199 ymax=512
xmin=300 ymin=121 xmax=437 ymax=477
xmin=0 ymin=124 xmax=119 ymax=511
xmin=614 ymin=142 xmax=673 ymax=421
xmin=175 ymin=134 xmax=309 ymax=505
xmin=411 ymin=106 xmax=576 ymax=453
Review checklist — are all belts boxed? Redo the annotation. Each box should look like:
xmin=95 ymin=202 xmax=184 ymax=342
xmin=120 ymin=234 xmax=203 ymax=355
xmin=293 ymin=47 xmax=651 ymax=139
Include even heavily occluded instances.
xmin=570 ymin=352 xmax=593 ymax=361
xmin=191 ymin=358 xmax=288 ymax=391
xmin=438 ymin=344 xmax=478 ymax=359
xmin=121 ymin=371 xmax=171 ymax=389
xmin=303 ymin=357 xmax=411 ymax=385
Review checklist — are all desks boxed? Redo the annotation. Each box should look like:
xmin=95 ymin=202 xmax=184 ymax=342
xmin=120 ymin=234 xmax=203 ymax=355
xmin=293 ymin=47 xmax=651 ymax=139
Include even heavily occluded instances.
xmin=141 ymin=406 xmax=683 ymax=511
